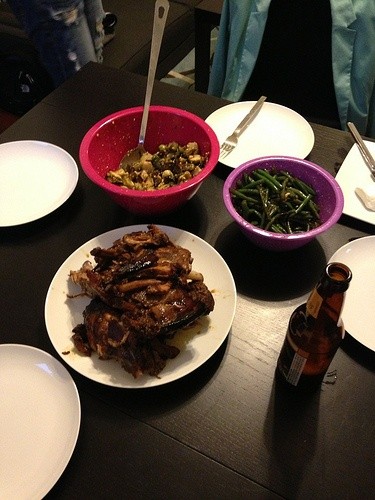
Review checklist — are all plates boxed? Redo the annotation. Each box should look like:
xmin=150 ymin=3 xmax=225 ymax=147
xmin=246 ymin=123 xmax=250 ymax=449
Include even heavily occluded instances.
xmin=328 ymin=235 xmax=375 ymax=352
xmin=335 ymin=140 xmax=375 ymax=225
xmin=45 ymin=225 xmax=236 ymax=388
xmin=0 ymin=344 xmax=81 ymax=500
xmin=0 ymin=141 xmax=79 ymax=227
xmin=204 ymin=101 xmax=315 ymax=169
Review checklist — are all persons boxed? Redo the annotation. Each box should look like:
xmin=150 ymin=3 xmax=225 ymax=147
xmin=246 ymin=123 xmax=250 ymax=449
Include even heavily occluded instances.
xmin=5 ymin=0 xmax=104 ymax=90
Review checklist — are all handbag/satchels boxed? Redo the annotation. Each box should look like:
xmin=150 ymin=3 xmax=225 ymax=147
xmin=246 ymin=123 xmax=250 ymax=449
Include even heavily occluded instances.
xmin=0 ymin=33 xmax=54 ymax=117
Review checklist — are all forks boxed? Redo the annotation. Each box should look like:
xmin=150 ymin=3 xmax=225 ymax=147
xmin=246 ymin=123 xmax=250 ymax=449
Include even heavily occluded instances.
xmin=221 ymin=96 xmax=266 ymax=152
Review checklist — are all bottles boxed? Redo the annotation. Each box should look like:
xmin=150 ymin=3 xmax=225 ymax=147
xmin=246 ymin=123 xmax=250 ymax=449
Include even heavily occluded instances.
xmin=277 ymin=262 xmax=352 ymax=395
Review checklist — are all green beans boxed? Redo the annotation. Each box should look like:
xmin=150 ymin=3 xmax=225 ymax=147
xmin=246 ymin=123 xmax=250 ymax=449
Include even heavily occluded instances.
xmin=231 ymin=169 xmax=321 ymax=234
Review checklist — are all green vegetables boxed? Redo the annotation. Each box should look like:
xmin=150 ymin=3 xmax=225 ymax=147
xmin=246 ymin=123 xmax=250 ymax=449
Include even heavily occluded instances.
xmin=151 ymin=143 xmax=191 ymax=180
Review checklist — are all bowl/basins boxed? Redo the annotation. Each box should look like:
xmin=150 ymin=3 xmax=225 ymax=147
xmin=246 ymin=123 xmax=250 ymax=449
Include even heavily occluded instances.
xmin=79 ymin=106 xmax=220 ymax=214
xmin=223 ymin=155 xmax=345 ymax=249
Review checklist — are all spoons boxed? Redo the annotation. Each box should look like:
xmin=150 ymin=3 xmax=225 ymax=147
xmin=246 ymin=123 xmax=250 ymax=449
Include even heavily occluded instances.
xmin=120 ymin=0 xmax=170 ymax=163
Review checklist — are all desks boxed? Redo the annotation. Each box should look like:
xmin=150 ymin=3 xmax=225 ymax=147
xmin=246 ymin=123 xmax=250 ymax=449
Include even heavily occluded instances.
xmin=0 ymin=56 xmax=374 ymax=500
